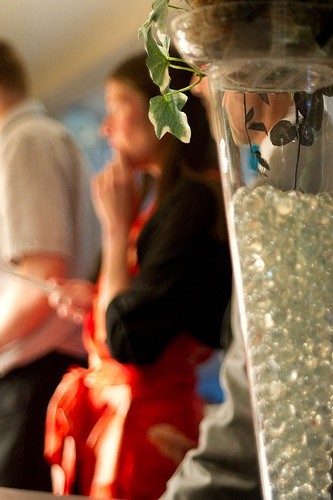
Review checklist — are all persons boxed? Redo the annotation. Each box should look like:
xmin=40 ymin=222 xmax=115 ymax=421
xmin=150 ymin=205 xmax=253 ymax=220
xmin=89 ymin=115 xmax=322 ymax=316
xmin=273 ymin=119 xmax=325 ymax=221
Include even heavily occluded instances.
xmin=0 ymin=38 xmax=101 ymax=494
xmin=159 ymin=61 xmax=332 ymax=500
xmin=223 ymin=62 xmax=332 ymax=195
xmin=45 ymin=43 xmax=233 ymax=500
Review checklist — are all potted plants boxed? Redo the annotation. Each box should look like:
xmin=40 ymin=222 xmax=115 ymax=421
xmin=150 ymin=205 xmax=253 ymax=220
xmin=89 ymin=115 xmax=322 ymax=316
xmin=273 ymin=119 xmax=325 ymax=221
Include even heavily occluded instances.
xmin=141 ymin=0 xmax=333 ymax=144
